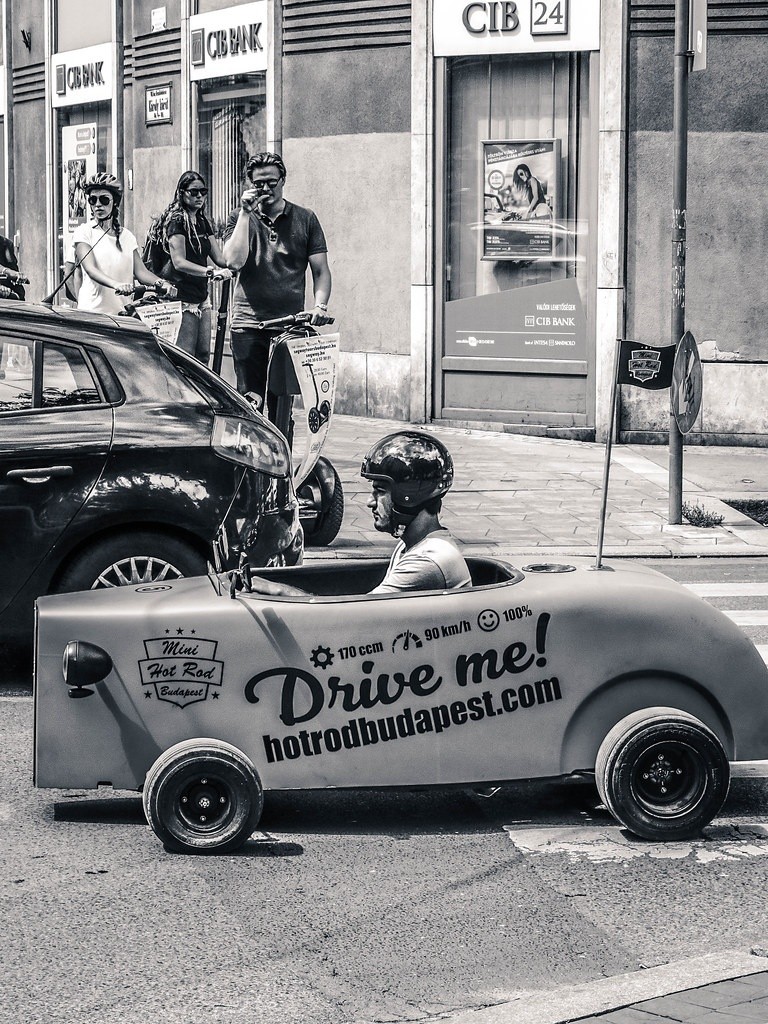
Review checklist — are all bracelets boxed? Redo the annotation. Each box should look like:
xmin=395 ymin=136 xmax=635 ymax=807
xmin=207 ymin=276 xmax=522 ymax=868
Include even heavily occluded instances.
xmin=154 ymin=279 xmax=166 ymax=287
xmin=2 ymin=268 xmax=10 ymax=274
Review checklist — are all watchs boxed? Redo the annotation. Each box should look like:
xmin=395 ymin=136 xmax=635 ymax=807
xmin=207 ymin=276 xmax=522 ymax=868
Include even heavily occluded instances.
xmin=206 ymin=265 xmax=213 ymax=278
xmin=314 ymin=304 xmax=329 ymax=311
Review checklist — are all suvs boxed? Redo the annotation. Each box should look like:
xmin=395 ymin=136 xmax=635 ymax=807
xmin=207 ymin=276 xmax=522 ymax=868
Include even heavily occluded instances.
xmin=0 ymin=298 xmax=304 ymax=663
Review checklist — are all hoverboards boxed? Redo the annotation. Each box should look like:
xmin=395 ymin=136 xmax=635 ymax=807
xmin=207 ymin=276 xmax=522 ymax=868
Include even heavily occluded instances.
xmin=302 ymin=362 xmax=332 ymax=434
xmin=257 ymin=309 xmax=345 ymax=548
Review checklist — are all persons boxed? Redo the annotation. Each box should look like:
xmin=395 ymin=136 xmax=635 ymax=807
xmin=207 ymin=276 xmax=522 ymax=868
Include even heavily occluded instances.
xmin=241 ymin=431 xmax=472 ymax=596
xmin=0 ymin=234 xmax=27 ymax=301
xmin=513 ymin=164 xmax=551 ymax=220
xmin=73 ymin=171 xmax=177 ymax=317
xmin=223 ymin=152 xmax=332 ymax=452
xmin=139 ymin=171 xmax=233 ymax=368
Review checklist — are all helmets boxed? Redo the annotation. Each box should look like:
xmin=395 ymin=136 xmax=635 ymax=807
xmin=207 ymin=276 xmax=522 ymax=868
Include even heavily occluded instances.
xmin=85 ymin=172 xmax=122 ymax=195
xmin=361 ymin=431 xmax=453 ymax=509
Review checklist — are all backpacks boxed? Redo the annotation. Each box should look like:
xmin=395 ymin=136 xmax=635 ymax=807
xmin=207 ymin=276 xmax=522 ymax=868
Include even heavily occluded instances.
xmin=142 ymin=205 xmax=191 ymax=275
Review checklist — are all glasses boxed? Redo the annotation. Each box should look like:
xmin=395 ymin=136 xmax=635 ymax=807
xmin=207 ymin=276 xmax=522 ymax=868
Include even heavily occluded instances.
xmin=250 ymin=175 xmax=281 ymax=189
xmin=87 ymin=196 xmax=115 ymax=206
xmin=184 ymin=188 xmax=208 ymax=196
